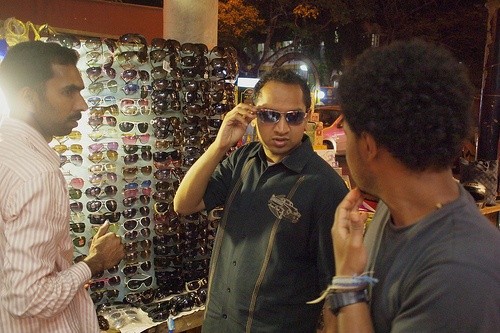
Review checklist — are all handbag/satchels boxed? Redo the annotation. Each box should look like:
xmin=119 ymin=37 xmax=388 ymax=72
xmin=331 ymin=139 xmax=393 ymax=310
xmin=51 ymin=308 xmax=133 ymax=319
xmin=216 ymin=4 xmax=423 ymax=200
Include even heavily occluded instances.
xmin=457 ymin=155 xmax=498 ymax=206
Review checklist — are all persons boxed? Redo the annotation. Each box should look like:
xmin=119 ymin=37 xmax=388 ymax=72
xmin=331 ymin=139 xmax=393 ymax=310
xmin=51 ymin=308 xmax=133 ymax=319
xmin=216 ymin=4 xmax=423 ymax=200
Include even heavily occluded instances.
xmin=174 ymin=70 xmax=350 ymax=333
xmin=0 ymin=40 xmax=125 ymax=333
xmin=332 ymin=38 xmax=500 ymax=333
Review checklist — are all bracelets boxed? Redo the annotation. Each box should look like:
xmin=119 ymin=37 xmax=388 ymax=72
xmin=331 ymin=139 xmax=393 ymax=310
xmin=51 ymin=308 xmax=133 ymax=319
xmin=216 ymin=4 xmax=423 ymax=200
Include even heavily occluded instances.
xmin=328 ymin=274 xmax=372 ymax=311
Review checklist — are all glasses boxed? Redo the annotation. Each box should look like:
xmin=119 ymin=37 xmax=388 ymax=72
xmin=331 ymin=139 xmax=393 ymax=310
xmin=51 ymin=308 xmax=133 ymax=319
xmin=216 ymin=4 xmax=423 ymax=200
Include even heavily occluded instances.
xmin=251 ymin=108 xmax=311 ymax=124
xmin=6 ymin=18 xmax=238 ymax=331
